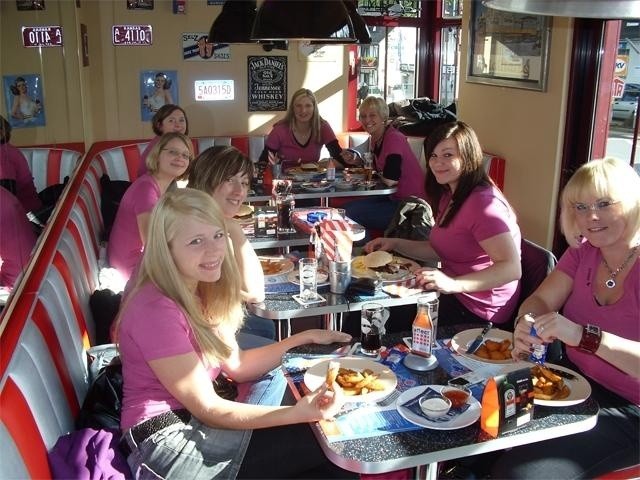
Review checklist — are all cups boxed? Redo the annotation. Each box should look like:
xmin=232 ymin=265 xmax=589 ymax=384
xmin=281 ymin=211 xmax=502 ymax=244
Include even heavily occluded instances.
xmin=417 ymin=296 xmax=441 ymax=347
xmin=299 ymin=258 xmax=317 ymax=300
xmin=360 ymin=302 xmax=384 ymax=357
xmin=328 ymin=260 xmax=351 ymax=294
xmin=330 ymin=209 xmax=346 ymax=220
xmin=271 ymin=164 xmax=282 ymax=179
xmin=276 ymin=192 xmax=296 ymax=232
xmin=361 ymin=159 xmax=373 ymax=184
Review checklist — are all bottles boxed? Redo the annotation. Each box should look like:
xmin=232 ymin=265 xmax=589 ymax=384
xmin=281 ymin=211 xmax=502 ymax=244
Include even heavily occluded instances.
xmin=328 ymin=157 xmax=335 ymax=180
xmin=412 ymin=303 xmax=432 ymax=357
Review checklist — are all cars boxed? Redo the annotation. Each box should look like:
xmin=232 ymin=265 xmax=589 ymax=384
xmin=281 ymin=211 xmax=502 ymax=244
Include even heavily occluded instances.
xmin=612 ymin=90 xmax=640 ymax=120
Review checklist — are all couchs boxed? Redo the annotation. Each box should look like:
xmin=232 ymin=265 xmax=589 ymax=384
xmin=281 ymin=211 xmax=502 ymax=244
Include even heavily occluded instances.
xmin=1 ymin=130 xmax=507 ymax=479
xmin=0 ymin=141 xmax=85 ymax=331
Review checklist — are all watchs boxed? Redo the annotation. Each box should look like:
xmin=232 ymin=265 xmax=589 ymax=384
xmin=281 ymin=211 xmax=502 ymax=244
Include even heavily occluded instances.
xmin=576 ymin=322 xmax=603 ymax=355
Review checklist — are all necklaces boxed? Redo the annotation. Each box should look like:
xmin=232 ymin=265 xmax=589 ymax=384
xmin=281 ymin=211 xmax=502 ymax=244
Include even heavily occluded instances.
xmin=593 ymin=244 xmax=640 ymax=288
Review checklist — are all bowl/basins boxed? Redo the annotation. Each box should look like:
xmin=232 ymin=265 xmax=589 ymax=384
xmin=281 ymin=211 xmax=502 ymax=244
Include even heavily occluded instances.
xmin=441 ymin=384 xmax=473 ymax=409
xmin=418 ymin=394 xmax=452 ymax=420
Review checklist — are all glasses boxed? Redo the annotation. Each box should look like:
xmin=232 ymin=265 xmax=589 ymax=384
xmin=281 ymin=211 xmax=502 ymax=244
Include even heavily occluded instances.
xmin=568 ymin=199 xmax=621 ymax=214
xmin=161 ymin=147 xmax=191 ymax=161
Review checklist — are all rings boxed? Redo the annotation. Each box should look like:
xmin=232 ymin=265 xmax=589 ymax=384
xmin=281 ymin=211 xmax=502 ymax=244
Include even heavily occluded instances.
xmin=539 ymin=325 xmax=546 ymax=332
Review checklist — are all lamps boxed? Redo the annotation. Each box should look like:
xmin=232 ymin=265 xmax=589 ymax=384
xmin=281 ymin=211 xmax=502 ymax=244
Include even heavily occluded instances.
xmin=310 ymin=1 xmax=373 ymax=44
xmin=250 ymin=0 xmax=358 ymax=42
xmin=206 ymin=1 xmax=288 ymax=45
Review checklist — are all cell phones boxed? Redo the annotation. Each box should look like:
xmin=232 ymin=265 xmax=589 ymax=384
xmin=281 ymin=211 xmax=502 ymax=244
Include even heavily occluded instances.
xmin=448 ymin=371 xmax=485 ymax=387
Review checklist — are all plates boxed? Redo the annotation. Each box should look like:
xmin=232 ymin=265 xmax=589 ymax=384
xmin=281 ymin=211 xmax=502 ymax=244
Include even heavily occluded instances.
xmin=350 ymin=255 xmax=421 ymax=284
xmin=343 ymin=167 xmax=367 ymax=180
xmin=287 ymin=269 xmax=329 ymax=287
xmin=451 ymin=327 xmax=515 ymax=366
xmin=284 ymin=167 xmax=328 ymax=177
xmin=500 ymin=361 xmax=592 ymax=407
xmin=304 ymin=357 xmax=398 ymax=404
xmin=396 ymin=384 xmax=481 ymax=431
xmin=300 ymin=183 xmax=333 ymax=192
xmin=257 ymin=256 xmax=295 ymax=278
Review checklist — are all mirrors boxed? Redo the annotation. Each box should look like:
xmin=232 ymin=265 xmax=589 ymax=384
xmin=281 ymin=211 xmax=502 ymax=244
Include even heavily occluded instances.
xmin=0 ymin=1 xmax=86 ymax=332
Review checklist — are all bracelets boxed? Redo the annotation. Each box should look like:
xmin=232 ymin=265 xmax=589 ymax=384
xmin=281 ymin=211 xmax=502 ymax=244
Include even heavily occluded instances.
xmin=513 ymin=311 xmax=535 ymax=328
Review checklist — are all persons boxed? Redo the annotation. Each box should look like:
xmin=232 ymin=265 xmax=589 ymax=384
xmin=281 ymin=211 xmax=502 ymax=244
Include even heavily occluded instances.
xmin=143 ymin=73 xmax=174 ymax=115
xmin=494 ymin=155 xmax=639 ymax=480
xmin=9 ymin=77 xmax=40 ymax=121
xmin=107 ymin=133 xmax=194 ymax=280
xmin=358 ymin=121 xmax=523 ymax=328
xmin=338 ymin=95 xmax=430 ymax=247
xmin=118 ymin=145 xmax=266 ymax=306
xmin=0 ymin=115 xmax=46 ymax=213
xmin=256 ymin=88 xmax=352 ymax=169
xmin=0 ymin=186 xmax=38 ymax=305
xmin=113 ymin=187 xmax=353 ymax=480
xmin=136 ymin=103 xmax=190 ymax=176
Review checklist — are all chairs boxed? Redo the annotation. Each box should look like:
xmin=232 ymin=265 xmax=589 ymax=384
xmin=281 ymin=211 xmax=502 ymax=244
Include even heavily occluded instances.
xmin=517 ymin=240 xmax=563 ymax=365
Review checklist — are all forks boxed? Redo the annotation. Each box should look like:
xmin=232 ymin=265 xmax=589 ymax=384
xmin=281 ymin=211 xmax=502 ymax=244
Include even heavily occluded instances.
xmin=326 ymin=389 xmax=401 ymax=422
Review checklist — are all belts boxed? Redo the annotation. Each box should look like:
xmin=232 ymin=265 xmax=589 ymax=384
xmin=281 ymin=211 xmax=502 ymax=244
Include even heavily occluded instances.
xmin=115 ymin=408 xmax=193 ymax=458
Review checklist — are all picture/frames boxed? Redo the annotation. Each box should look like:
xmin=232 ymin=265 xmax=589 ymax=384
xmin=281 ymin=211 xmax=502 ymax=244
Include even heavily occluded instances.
xmin=466 ymin=2 xmax=552 ymax=93
xmin=1 ymin=73 xmax=48 ymax=128
xmin=137 ymin=68 xmax=181 ymax=124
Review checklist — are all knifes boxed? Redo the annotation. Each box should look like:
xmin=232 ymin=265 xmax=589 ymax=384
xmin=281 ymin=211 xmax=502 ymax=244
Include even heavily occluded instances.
xmin=464 ymin=322 xmax=494 ymax=357
